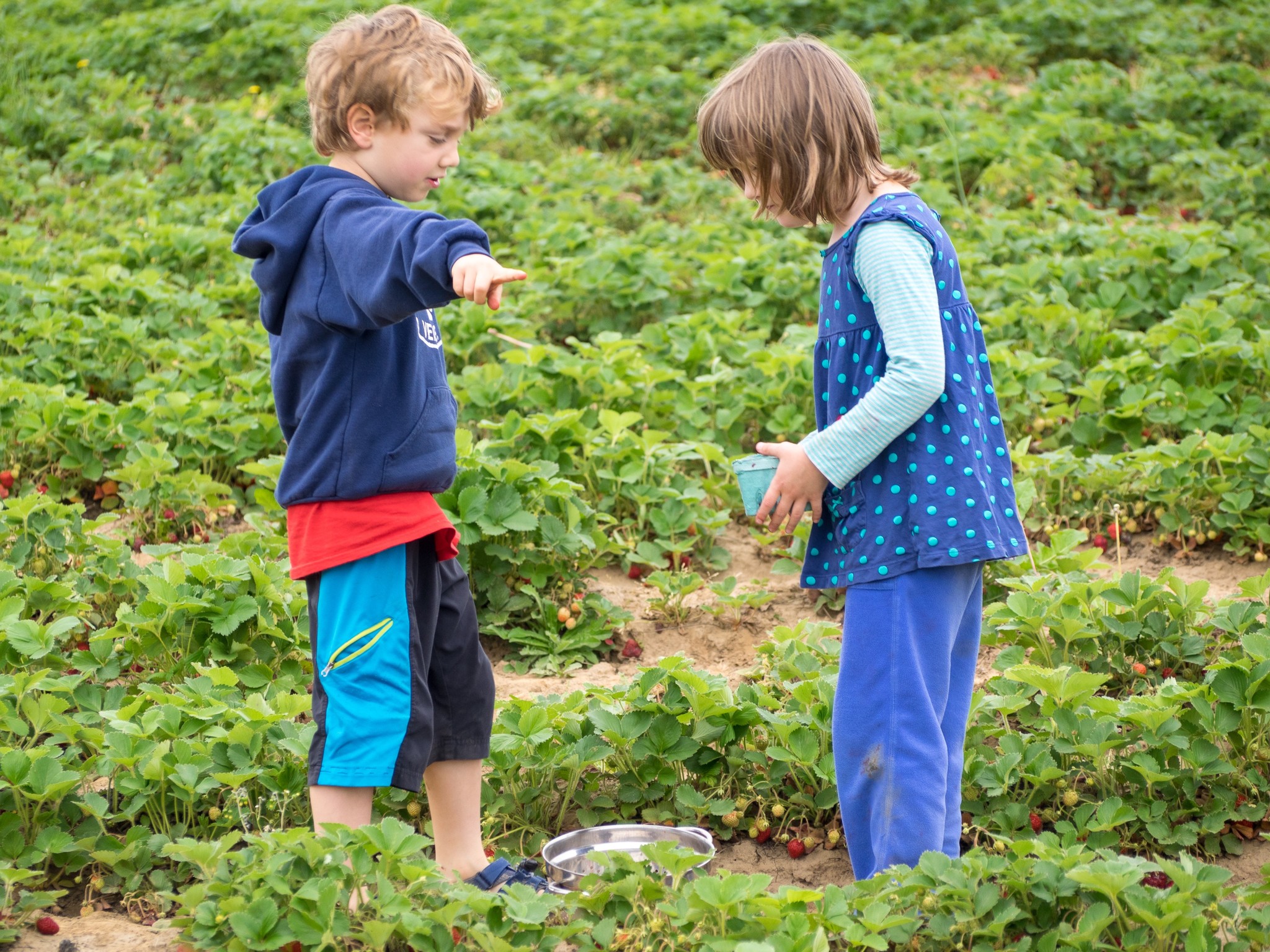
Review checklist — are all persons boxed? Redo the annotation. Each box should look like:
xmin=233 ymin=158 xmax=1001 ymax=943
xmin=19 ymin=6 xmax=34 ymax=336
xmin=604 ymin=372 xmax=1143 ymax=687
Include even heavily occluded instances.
xmin=694 ymin=40 xmax=1028 ymax=947
xmin=233 ymin=6 xmax=556 ymax=914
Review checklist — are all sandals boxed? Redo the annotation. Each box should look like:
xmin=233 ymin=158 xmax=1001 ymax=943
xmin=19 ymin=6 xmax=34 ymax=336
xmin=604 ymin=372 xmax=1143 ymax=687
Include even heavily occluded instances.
xmin=448 ymin=857 xmax=589 ymax=921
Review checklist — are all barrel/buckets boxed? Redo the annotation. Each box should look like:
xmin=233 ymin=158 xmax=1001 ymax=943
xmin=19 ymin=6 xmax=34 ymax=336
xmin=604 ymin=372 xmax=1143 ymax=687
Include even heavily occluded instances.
xmin=542 ymin=824 xmax=715 ymax=922
